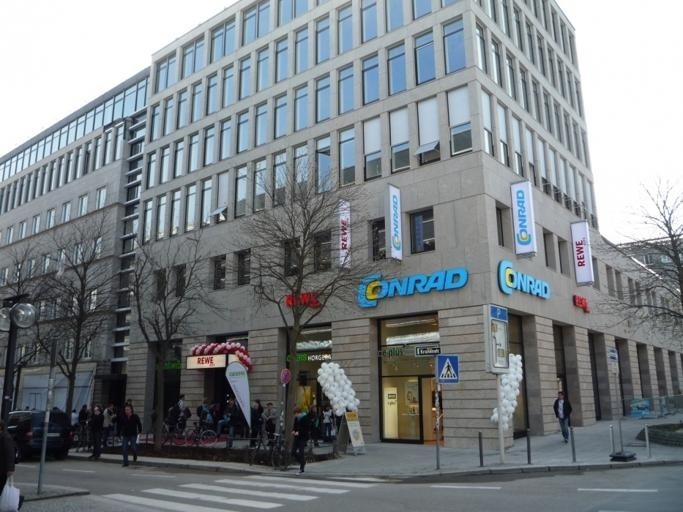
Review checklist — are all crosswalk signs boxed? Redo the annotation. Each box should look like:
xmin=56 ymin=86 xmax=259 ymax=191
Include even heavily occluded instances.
xmin=435 ymin=354 xmax=461 ymax=384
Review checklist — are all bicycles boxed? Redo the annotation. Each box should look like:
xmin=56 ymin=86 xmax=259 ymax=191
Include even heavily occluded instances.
xmin=250 ymin=433 xmax=290 ymax=470
xmin=153 ymin=422 xmax=218 ymax=446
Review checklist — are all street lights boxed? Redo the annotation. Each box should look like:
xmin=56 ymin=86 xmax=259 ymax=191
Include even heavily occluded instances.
xmin=0 ymin=294 xmax=37 ymax=423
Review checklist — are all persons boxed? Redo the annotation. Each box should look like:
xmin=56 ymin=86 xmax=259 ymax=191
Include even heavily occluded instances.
xmin=0 ymin=419 xmax=15 ymax=495
xmin=552 ymin=391 xmax=571 ymax=441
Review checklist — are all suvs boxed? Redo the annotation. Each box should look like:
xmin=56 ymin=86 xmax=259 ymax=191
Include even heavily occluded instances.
xmin=8 ymin=411 xmax=74 ymax=463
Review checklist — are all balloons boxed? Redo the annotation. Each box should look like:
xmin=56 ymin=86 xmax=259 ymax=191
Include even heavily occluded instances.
xmin=491 ymin=353 xmax=523 ymax=431
xmin=315 ymin=361 xmax=359 ymax=416
xmin=189 ymin=341 xmax=253 ymax=372
